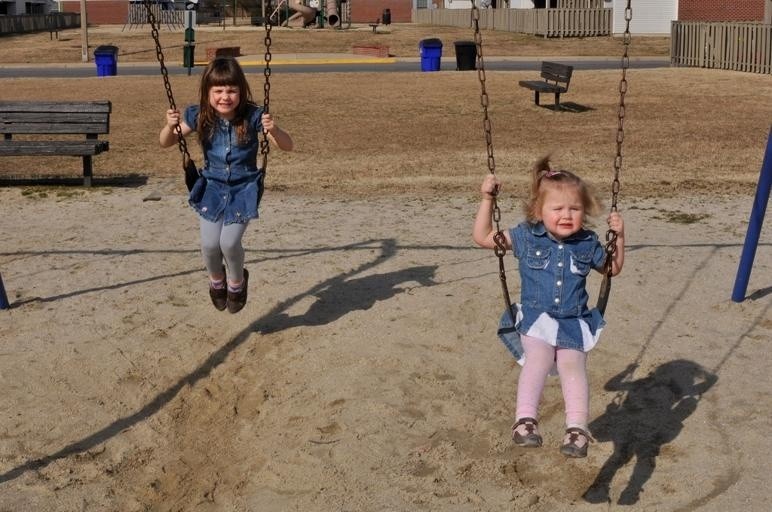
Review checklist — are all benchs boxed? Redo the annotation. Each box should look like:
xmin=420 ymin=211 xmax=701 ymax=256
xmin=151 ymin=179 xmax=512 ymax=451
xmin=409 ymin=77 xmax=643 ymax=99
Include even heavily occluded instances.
xmin=196 ymin=14 xmax=224 ymax=30
xmin=202 ymin=45 xmax=242 ymax=63
xmin=350 ymin=43 xmax=392 ymax=60
xmin=518 ymin=58 xmax=574 ymax=113
xmin=368 ymin=18 xmax=381 ymax=33
xmin=0 ymin=97 xmax=117 ymax=187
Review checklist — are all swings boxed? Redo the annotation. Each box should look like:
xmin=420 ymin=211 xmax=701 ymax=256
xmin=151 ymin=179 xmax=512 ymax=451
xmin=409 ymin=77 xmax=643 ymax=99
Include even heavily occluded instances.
xmin=143 ymin=0 xmax=272 ymax=209
xmin=471 ymin=0 xmax=632 ymax=328
xmin=588 ymin=246 xmax=771 ymax=442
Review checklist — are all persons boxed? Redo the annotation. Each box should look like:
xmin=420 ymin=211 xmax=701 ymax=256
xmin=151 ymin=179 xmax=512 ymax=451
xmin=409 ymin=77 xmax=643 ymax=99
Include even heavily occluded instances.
xmin=473 ymin=153 xmax=624 ymax=459
xmin=159 ymin=56 xmax=292 ymax=313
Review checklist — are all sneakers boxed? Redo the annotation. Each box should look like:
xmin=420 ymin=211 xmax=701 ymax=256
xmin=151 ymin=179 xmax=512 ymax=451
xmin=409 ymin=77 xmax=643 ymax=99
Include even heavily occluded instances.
xmin=560 ymin=427 xmax=594 ymax=457
xmin=226 ymin=268 xmax=249 ymax=313
xmin=209 ymin=264 xmax=226 ymax=311
xmin=512 ymin=417 xmax=543 ymax=448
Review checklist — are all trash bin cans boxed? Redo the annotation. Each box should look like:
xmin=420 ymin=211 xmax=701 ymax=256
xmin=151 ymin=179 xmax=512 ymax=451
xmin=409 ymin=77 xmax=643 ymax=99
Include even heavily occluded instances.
xmin=453 ymin=40 xmax=477 ymax=71
xmin=93 ymin=45 xmax=119 ymax=76
xmin=419 ymin=38 xmax=443 ymax=72
xmin=383 ymin=8 xmax=391 ymax=24
